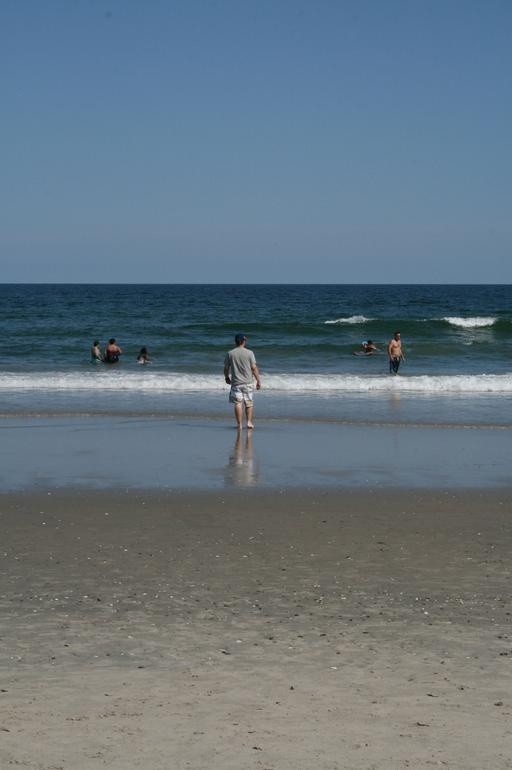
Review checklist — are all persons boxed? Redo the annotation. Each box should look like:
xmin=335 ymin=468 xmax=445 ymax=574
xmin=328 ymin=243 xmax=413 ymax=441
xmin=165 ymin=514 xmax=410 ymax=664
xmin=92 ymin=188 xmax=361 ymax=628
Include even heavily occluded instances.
xmin=89 ymin=338 xmax=104 ymax=364
xmin=386 ymin=330 xmax=405 ymax=377
xmin=224 ymin=428 xmax=260 ymax=488
xmin=223 ymin=333 xmax=262 ymax=431
xmin=137 ymin=346 xmax=152 ymax=364
xmin=102 ymin=338 xmax=122 ymax=364
xmin=362 ymin=339 xmax=376 ymax=355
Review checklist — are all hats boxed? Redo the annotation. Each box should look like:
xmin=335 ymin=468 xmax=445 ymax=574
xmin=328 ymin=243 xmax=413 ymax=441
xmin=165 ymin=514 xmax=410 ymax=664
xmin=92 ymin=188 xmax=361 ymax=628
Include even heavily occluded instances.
xmin=235 ymin=334 xmax=247 ymax=341
